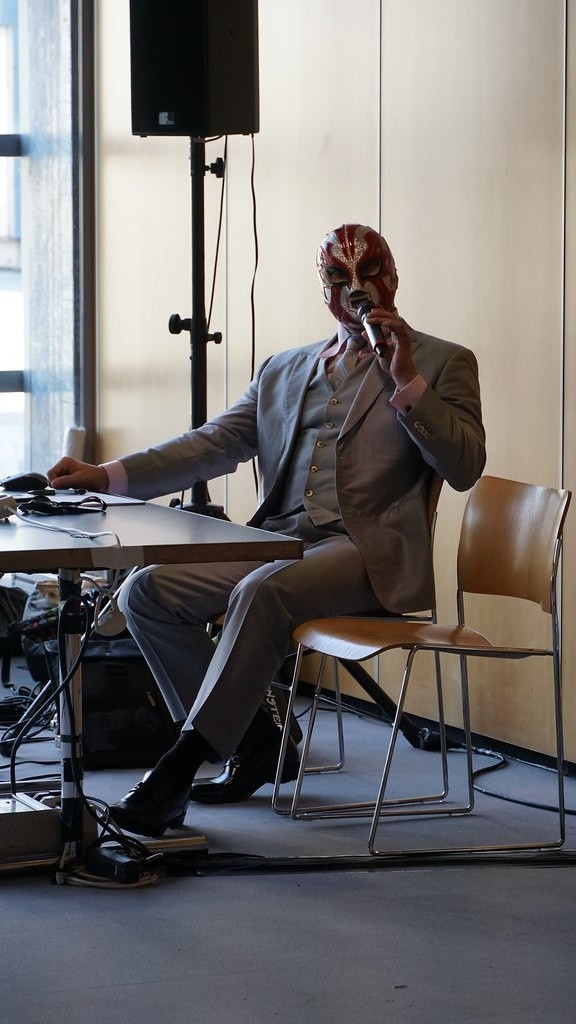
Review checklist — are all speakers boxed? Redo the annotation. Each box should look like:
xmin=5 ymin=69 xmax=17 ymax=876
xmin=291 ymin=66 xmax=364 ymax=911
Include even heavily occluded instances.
xmin=130 ymin=0 xmax=260 ymax=138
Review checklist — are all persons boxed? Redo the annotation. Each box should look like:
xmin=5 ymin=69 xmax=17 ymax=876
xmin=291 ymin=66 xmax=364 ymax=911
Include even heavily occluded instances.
xmin=47 ymin=224 xmax=487 ymax=838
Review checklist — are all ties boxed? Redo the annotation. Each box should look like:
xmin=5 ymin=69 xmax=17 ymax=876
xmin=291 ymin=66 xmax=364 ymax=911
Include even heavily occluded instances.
xmin=330 ymin=335 xmax=367 ymax=393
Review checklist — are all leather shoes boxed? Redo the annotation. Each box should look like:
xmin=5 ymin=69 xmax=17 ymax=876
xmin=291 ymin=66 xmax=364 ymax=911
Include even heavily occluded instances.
xmin=106 ymin=777 xmax=193 ymax=839
xmin=191 ymin=728 xmax=302 ymax=803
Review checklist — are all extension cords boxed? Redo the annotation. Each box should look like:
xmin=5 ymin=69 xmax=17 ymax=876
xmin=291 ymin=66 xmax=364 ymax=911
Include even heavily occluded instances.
xmin=0 ymin=495 xmax=17 ymax=519
xmin=88 ymin=849 xmax=140 ymax=888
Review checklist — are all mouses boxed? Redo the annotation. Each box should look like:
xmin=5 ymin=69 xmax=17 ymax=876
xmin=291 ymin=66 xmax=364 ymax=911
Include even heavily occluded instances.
xmin=0 ymin=472 xmax=48 ymax=491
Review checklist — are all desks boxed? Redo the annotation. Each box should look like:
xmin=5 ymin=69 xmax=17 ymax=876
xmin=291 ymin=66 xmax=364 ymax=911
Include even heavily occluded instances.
xmin=0 ymin=488 xmax=304 ymax=874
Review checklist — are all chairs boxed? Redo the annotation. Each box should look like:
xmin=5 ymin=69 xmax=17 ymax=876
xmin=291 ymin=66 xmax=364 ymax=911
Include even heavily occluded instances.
xmin=193 ymin=512 xmax=449 ymax=816
xmin=269 ymin=475 xmax=576 ymax=856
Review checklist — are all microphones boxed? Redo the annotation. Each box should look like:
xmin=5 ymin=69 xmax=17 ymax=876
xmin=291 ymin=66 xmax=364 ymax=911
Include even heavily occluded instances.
xmin=358 ymin=300 xmax=388 ymax=358
